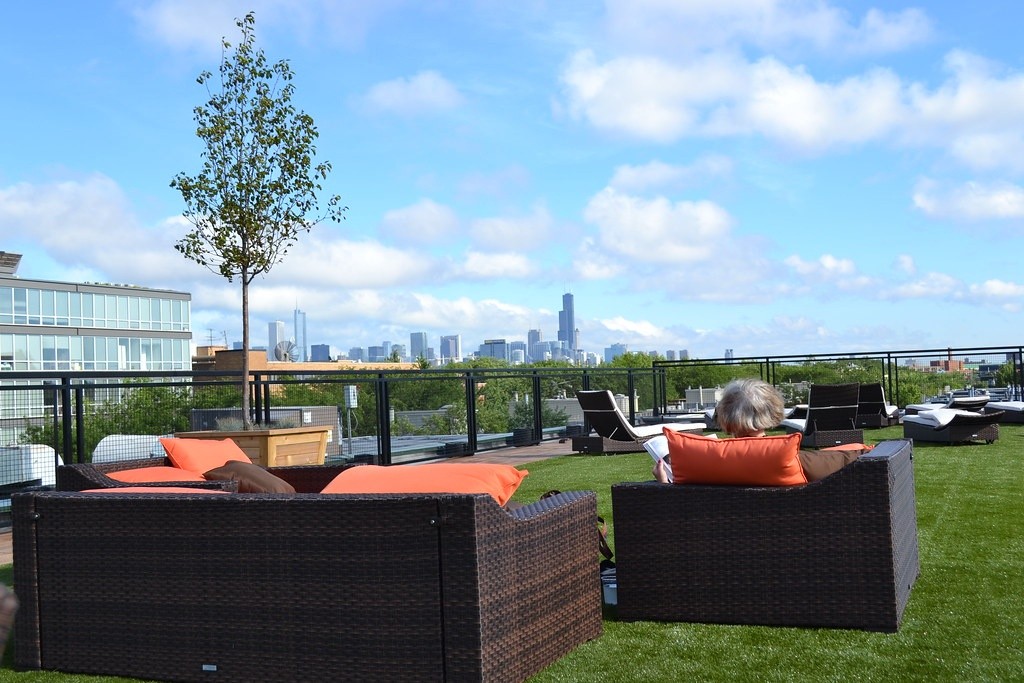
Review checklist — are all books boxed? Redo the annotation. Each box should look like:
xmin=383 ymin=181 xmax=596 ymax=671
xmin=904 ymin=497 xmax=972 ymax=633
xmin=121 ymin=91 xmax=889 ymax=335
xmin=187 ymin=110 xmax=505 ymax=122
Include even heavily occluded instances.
xmin=643 ymin=433 xmax=719 ymax=483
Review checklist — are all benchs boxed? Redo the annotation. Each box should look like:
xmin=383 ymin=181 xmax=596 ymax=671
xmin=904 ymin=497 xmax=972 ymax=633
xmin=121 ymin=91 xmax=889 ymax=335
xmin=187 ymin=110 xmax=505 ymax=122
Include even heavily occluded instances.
xmin=572 ymin=381 xmax=1024 ymax=455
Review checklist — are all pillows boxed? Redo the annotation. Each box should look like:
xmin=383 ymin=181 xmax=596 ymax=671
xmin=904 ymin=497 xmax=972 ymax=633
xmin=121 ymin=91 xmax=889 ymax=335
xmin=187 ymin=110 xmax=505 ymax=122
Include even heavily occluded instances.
xmin=795 ymin=450 xmax=870 ymax=483
xmin=200 ymin=460 xmax=298 ymax=494
xmin=320 ymin=464 xmax=530 ymax=507
xmin=661 ymin=427 xmax=808 ymax=486
xmin=76 ymin=485 xmax=232 ymax=494
xmin=160 ymin=436 xmax=254 ymax=476
xmin=105 ymin=464 xmax=208 ymax=483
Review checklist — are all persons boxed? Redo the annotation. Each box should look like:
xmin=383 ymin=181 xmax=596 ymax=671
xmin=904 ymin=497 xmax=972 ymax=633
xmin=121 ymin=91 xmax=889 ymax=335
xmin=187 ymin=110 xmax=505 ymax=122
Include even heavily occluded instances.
xmin=652 ymin=378 xmax=785 ymax=484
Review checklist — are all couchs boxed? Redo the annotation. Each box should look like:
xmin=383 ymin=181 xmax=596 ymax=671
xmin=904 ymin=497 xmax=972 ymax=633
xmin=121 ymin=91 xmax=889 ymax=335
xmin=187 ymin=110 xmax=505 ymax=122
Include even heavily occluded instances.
xmin=55 ymin=454 xmax=370 ymax=497
xmin=613 ymin=438 xmax=923 ymax=632
xmin=11 ymin=486 xmax=605 ymax=683
